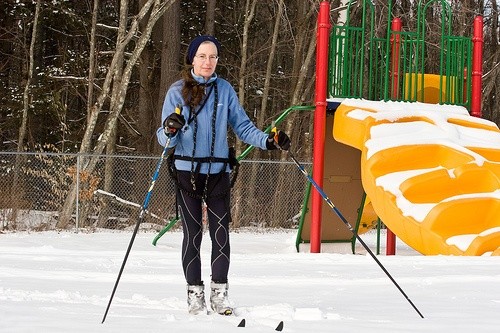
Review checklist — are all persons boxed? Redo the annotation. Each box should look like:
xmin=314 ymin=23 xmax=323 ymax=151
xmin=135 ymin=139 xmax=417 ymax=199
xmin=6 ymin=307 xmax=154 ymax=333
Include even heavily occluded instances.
xmin=156 ymin=33 xmax=290 ymax=317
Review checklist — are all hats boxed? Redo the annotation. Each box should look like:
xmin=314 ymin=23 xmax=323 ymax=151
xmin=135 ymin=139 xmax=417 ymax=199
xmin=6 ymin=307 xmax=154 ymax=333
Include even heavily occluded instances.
xmin=184 ymin=35 xmax=220 ymax=64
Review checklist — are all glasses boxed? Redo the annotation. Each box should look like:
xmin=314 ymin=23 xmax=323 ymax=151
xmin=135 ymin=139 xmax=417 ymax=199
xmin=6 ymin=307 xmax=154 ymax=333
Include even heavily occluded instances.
xmin=196 ymin=54 xmax=220 ymax=61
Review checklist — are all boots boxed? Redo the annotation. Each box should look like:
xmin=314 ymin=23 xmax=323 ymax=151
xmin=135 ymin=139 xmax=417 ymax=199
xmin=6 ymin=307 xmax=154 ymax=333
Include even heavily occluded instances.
xmin=210 ymin=282 xmax=233 ymax=315
xmin=186 ymin=284 xmax=208 ymax=316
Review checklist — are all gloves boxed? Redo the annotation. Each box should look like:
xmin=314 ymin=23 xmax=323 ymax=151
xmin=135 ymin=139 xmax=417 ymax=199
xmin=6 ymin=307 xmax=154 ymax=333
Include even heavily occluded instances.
xmin=266 ymin=128 xmax=291 ymax=150
xmin=163 ymin=112 xmax=186 ymax=137
